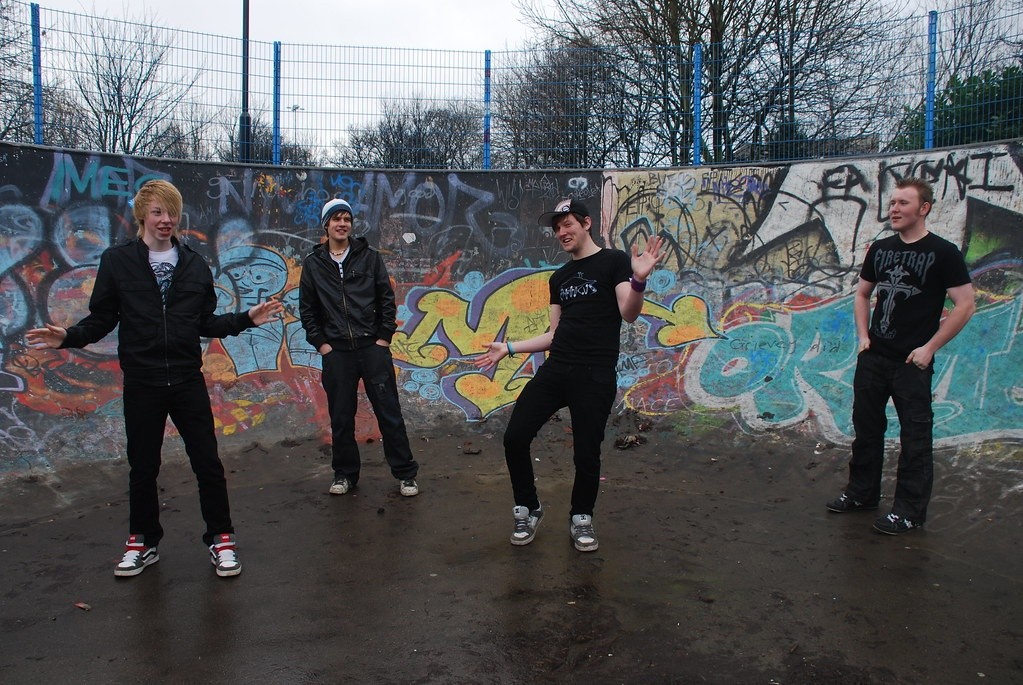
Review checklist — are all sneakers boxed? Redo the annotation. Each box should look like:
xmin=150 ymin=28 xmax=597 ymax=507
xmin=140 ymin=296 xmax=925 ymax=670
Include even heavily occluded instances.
xmin=510 ymin=501 xmax=545 ymax=545
xmin=825 ymin=491 xmax=881 ymax=513
xmin=568 ymin=514 xmax=598 ymax=552
xmin=209 ymin=533 xmax=241 ymax=577
xmin=113 ymin=533 xmax=159 ymax=576
xmin=398 ymin=478 xmax=418 ymax=496
xmin=328 ymin=476 xmax=353 ymax=495
xmin=873 ymin=512 xmax=923 ymax=535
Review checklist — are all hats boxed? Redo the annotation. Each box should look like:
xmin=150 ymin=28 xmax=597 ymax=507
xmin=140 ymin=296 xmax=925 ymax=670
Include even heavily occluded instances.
xmin=320 ymin=198 xmax=351 ymax=230
xmin=538 ymin=198 xmax=589 ymax=227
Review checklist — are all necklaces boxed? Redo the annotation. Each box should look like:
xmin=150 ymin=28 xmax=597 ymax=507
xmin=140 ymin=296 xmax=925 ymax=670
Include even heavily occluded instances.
xmin=328 ymin=246 xmax=348 ymax=256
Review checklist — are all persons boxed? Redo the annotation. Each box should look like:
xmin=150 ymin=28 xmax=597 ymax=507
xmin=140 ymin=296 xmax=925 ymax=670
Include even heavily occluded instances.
xmin=820 ymin=180 xmax=974 ymax=532
xmin=297 ymin=197 xmax=418 ymax=499
xmin=25 ymin=176 xmax=287 ymax=580
xmin=475 ymin=197 xmax=668 ymax=555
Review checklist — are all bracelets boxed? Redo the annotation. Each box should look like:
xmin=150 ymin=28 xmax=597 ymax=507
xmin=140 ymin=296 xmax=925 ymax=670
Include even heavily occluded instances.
xmin=505 ymin=341 xmax=516 ymax=359
xmin=630 ymin=274 xmax=649 ymax=293
